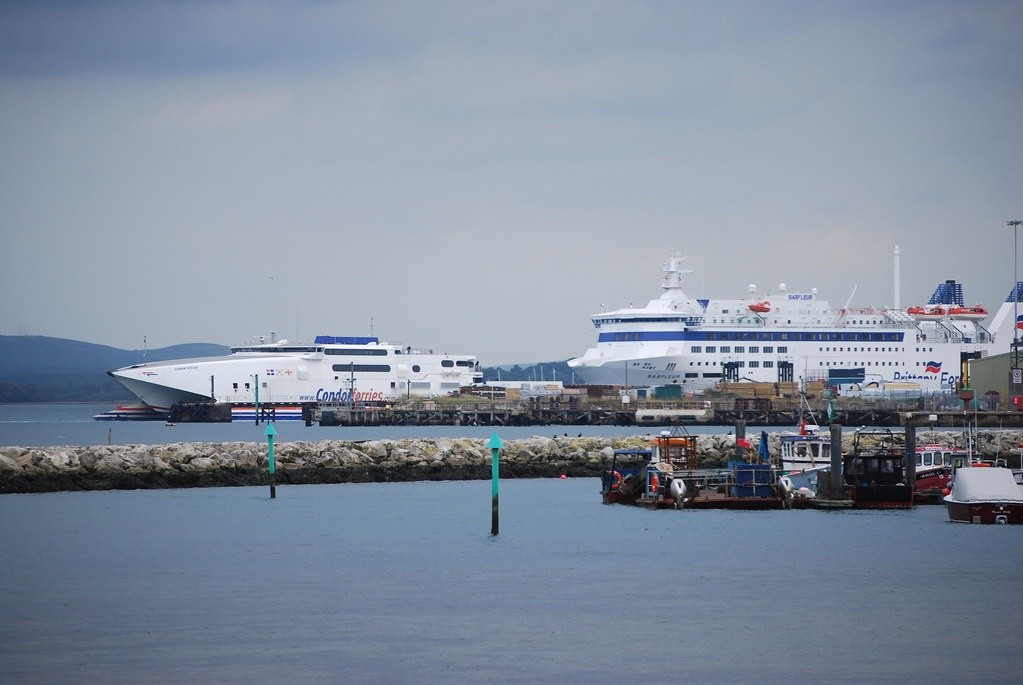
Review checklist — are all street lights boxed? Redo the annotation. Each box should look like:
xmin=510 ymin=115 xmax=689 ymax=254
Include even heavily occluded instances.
xmin=1007 ymin=217 xmax=1023 ymax=398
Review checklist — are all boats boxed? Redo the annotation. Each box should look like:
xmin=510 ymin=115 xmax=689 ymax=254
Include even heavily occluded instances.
xmin=905 ymin=306 xmax=948 ymax=321
xmin=748 ymin=303 xmax=772 ymax=313
xmin=942 ymin=465 xmax=1023 ymax=525
xmin=946 ymin=305 xmax=989 ymax=320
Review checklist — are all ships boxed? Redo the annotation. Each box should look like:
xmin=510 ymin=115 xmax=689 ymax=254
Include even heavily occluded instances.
xmin=102 ymin=330 xmax=481 ymax=419
xmin=564 ymin=252 xmax=1023 ymax=401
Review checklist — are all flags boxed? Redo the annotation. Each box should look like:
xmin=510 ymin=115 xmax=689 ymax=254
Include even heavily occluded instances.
xmin=737 ymin=438 xmax=751 ymax=448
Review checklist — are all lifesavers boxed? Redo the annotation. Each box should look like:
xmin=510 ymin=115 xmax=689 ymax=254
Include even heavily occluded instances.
xmin=652 ymin=475 xmax=659 ymax=492
xmin=608 ymin=471 xmax=622 ymax=489
